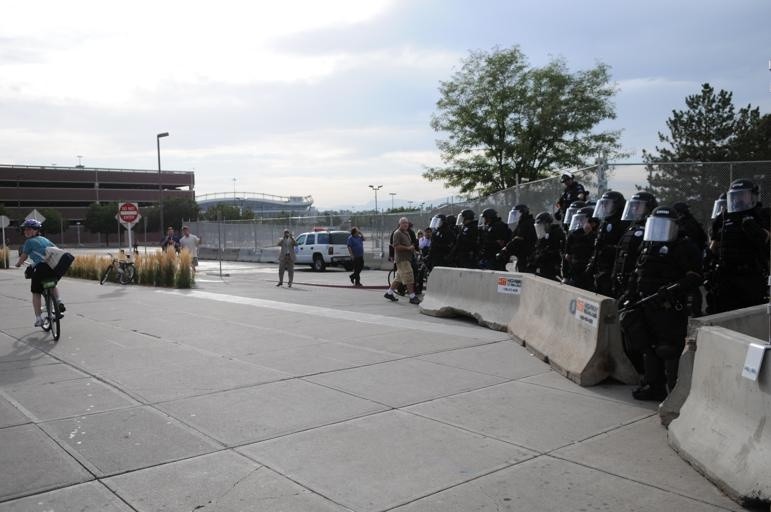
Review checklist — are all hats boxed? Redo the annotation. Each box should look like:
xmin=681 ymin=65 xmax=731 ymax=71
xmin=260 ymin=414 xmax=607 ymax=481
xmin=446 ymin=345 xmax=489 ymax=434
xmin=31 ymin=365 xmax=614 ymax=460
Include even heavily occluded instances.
xmin=560 ymin=172 xmax=575 ymax=182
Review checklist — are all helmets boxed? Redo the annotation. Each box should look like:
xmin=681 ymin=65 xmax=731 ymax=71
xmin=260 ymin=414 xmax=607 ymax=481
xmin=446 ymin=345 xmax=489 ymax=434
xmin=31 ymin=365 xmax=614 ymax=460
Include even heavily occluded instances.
xmin=564 ymin=192 xmax=679 ymax=242
xmin=428 ymin=205 xmax=554 ymax=239
xmin=20 ymin=219 xmax=42 ymax=230
xmin=712 ymin=178 xmax=762 ymax=218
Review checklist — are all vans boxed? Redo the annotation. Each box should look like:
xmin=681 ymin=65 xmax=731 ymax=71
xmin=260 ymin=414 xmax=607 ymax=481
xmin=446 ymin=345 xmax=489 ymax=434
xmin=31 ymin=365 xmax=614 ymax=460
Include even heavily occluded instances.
xmin=291 ymin=227 xmax=356 ymax=272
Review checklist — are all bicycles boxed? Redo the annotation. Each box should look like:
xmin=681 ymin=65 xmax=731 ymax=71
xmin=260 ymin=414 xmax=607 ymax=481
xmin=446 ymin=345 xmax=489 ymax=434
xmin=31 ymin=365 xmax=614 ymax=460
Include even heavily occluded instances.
xmin=100 ymin=253 xmax=136 ymax=284
xmin=386 ymin=263 xmax=430 ymax=295
xmin=22 ymin=262 xmax=64 ymax=341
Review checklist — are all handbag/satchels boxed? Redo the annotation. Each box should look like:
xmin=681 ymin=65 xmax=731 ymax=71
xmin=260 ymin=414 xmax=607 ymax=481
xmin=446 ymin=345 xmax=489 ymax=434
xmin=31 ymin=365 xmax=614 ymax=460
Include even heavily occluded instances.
xmin=31 ymin=236 xmax=75 ymax=281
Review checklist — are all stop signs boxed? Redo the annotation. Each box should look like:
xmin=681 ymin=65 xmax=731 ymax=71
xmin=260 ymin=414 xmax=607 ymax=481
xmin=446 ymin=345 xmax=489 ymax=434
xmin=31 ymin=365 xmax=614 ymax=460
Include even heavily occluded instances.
xmin=117 ymin=203 xmax=139 ymax=223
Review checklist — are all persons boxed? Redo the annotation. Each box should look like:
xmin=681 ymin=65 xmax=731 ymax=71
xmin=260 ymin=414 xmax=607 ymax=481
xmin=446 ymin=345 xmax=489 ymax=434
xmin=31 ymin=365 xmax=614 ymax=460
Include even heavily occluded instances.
xmin=180 ymin=227 xmax=200 ymax=283
xmin=15 ymin=217 xmax=66 ymax=327
xmin=160 ymin=226 xmax=181 ymax=253
xmin=276 ymin=230 xmax=297 ymax=287
xmin=347 ymin=226 xmax=365 ymax=287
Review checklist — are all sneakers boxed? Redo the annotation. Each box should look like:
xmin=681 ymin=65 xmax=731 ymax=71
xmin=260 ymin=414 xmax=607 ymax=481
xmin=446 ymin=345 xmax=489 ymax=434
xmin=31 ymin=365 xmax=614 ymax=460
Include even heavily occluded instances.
xmin=409 ymin=296 xmax=421 ymax=305
xmin=631 ymin=374 xmax=669 ymax=403
xmin=349 ymin=275 xmax=363 ymax=286
xmin=34 ymin=320 xmax=44 ymax=327
xmin=57 ymin=301 xmax=66 ymax=312
xmin=383 ymin=292 xmax=399 ymax=301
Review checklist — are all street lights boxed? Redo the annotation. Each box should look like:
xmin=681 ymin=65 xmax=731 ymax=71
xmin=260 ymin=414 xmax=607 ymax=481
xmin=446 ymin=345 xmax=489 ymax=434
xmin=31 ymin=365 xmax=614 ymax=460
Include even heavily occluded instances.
xmin=232 ymin=177 xmax=236 ymax=202
xmin=157 ymin=132 xmax=169 ymax=244
xmin=76 ymin=222 xmax=81 ymax=246
xmin=407 ymin=201 xmax=413 ymax=209
xmin=418 ymin=202 xmax=424 ymax=216
xmin=369 ymin=184 xmax=384 ymax=215
xmin=389 ymin=193 xmax=396 ymax=211
